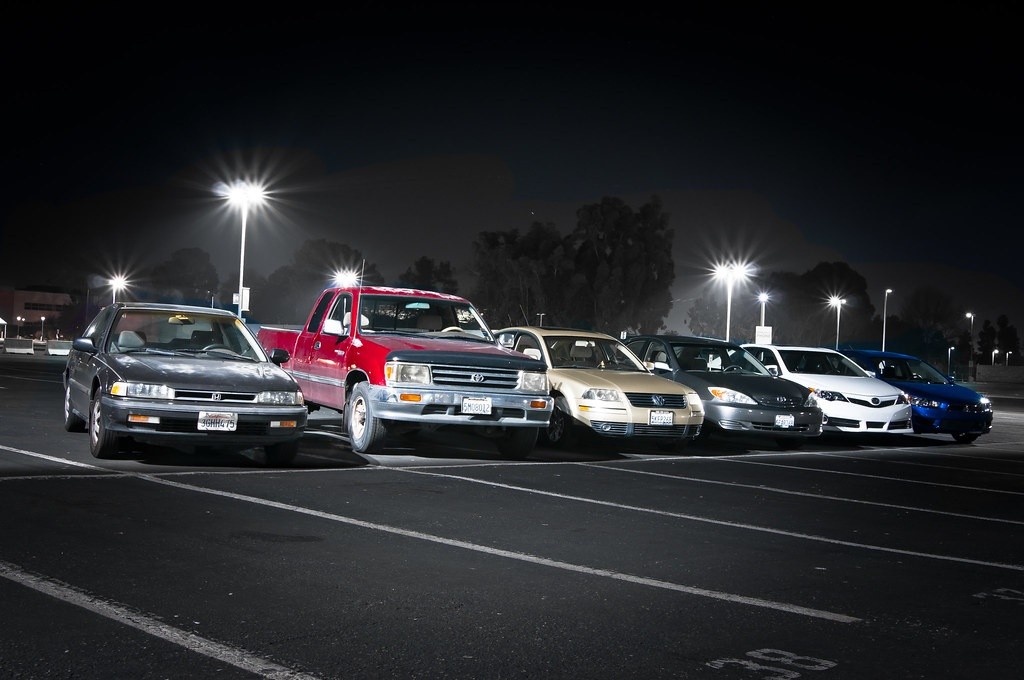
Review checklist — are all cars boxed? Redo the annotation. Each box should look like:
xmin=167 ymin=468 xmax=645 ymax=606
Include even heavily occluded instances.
xmin=611 ymin=335 xmax=824 ymax=438
xmin=62 ymin=302 xmax=307 ymax=464
xmin=490 ymin=326 xmax=705 ymax=445
xmin=832 ymin=348 xmax=994 ymax=444
xmin=706 ymin=342 xmax=914 ymax=433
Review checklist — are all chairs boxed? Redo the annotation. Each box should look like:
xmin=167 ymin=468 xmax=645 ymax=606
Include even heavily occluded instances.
xmin=563 ymin=344 xmax=596 ymax=367
xmin=691 ymin=358 xmax=708 ymax=370
xmin=416 ymin=314 xmax=442 ymax=332
xmin=188 ymin=330 xmax=218 ymax=349
xmin=882 ymin=366 xmax=896 ymax=379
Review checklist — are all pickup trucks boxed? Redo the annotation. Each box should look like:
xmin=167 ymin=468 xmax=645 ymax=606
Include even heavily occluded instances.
xmin=255 ymin=285 xmax=554 ymax=458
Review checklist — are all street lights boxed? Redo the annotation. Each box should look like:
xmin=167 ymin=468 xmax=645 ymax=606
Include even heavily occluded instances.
xmin=967 ymin=312 xmax=974 ymax=331
xmin=991 ymin=349 xmax=1012 ymax=365
xmin=948 ymin=347 xmax=955 ymax=376
xmin=759 ymin=293 xmax=767 ymax=326
xmin=17 ymin=316 xmax=21 ymax=338
xmin=882 ymin=288 xmax=893 ymax=352
xmin=41 ymin=317 xmax=45 ymax=336
xmin=228 ymin=183 xmax=264 ymax=320
xmin=336 ymin=272 xmax=358 ymax=315
xmin=829 ymin=297 xmax=841 ymax=349
xmin=716 ymin=263 xmax=748 ymax=342
xmin=112 ymin=276 xmax=124 ymax=303
